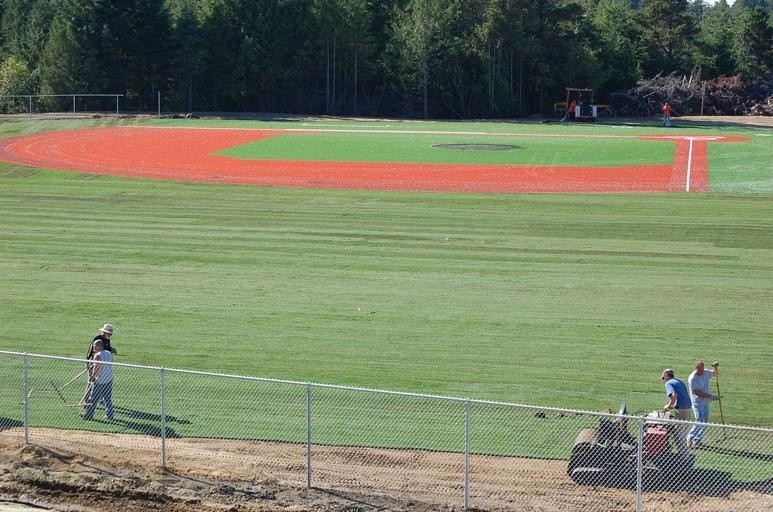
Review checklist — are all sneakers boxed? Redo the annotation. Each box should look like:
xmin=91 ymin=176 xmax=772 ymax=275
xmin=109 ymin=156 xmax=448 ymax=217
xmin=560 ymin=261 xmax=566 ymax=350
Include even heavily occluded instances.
xmin=78 ymin=410 xmax=113 ymax=420
xmin=686 ymin=439 xmax=705 ymax=450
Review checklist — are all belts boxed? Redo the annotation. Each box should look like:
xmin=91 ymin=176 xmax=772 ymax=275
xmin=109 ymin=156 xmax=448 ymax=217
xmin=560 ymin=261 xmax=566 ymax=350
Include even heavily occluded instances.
xmin=674 ymin=405 xmax=692 ymax=408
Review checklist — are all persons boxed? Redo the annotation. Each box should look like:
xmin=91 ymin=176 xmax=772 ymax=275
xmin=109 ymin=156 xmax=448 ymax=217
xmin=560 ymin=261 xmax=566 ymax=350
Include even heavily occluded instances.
xmin=659 ymin=368 xmax=695 ymax=454
xmin=77 ymin=339 xmax=114 ymax=419
xmin=567 ymin=98 xmax=577 ymax=120
xmin=686 ymin=360 xmax=723 ymax=446
xmin=81 ymin=323 xmax=118 ymax=409
xmin=661 ymin=102 xmax=671 ymax=123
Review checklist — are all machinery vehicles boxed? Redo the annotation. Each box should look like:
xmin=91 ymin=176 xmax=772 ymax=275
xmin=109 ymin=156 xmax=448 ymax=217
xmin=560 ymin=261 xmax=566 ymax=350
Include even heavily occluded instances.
xmin=553 ymin=88 xmax=616 ymax=122
xmin=567 ymin=402 xmax=694 ymax=487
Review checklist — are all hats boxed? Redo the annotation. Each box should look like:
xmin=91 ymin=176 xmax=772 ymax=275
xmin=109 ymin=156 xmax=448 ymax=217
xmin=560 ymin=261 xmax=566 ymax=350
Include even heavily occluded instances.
xmin=98 ymin=323 xmax=115 ymax=335
xmin=659 ymin=368 xmax=673 ymax=380
